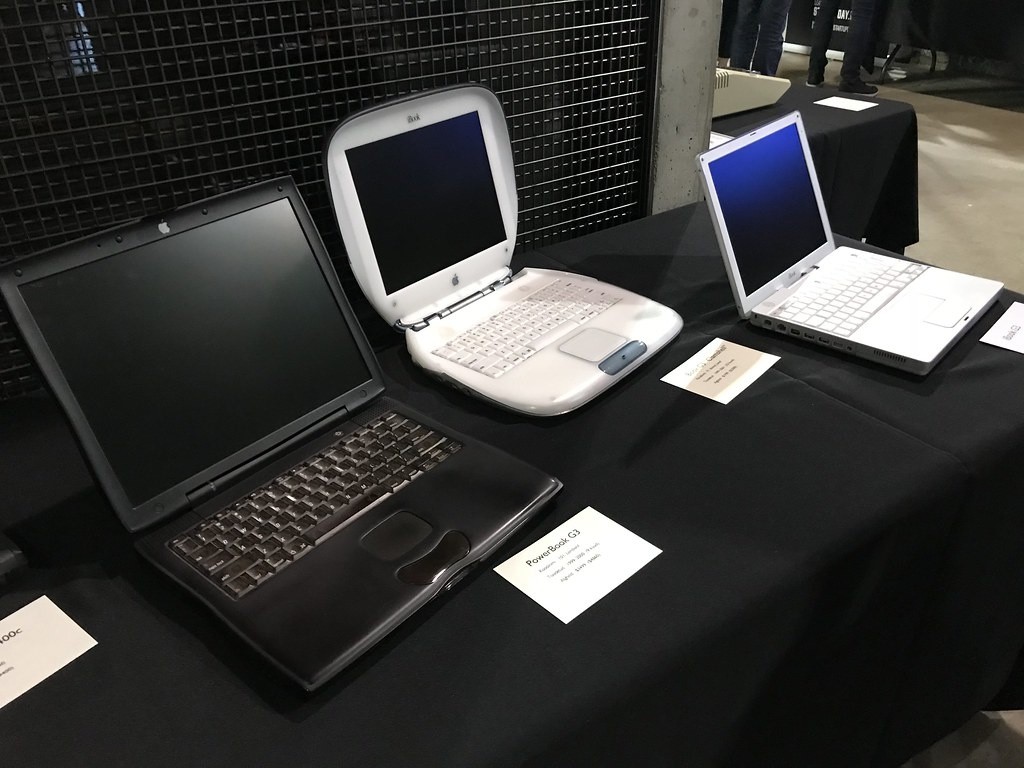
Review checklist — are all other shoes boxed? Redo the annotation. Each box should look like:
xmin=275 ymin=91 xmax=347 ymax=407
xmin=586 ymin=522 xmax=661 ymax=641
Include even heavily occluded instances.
xmin=805 ymin=76 xmax=825 ymax=87
xmin=838 ymin=83 xmax=879 ymax=97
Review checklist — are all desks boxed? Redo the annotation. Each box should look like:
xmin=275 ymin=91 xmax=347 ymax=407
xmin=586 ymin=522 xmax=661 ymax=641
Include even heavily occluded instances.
xmin=712 ymin=82 xmax=920 ymax=257
xmin=0 ymin=200 xmax=1024 ymax=768
xmin=876 ymin=0 xmax=1024 ymax=85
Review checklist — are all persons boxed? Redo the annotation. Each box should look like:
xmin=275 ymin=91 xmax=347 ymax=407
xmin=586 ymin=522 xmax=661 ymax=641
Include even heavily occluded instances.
xmin=719 ymin=0 xmax=879 ymax=95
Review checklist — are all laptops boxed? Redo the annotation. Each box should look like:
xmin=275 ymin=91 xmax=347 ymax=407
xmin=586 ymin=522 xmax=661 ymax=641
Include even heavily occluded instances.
xmin=325 ymin=83 xmax=684 ymax=415
xmin=694 ymin=109 xmax=1005 ymax=376
xmin=0 ymin=174 xmax=564 ymax=691
